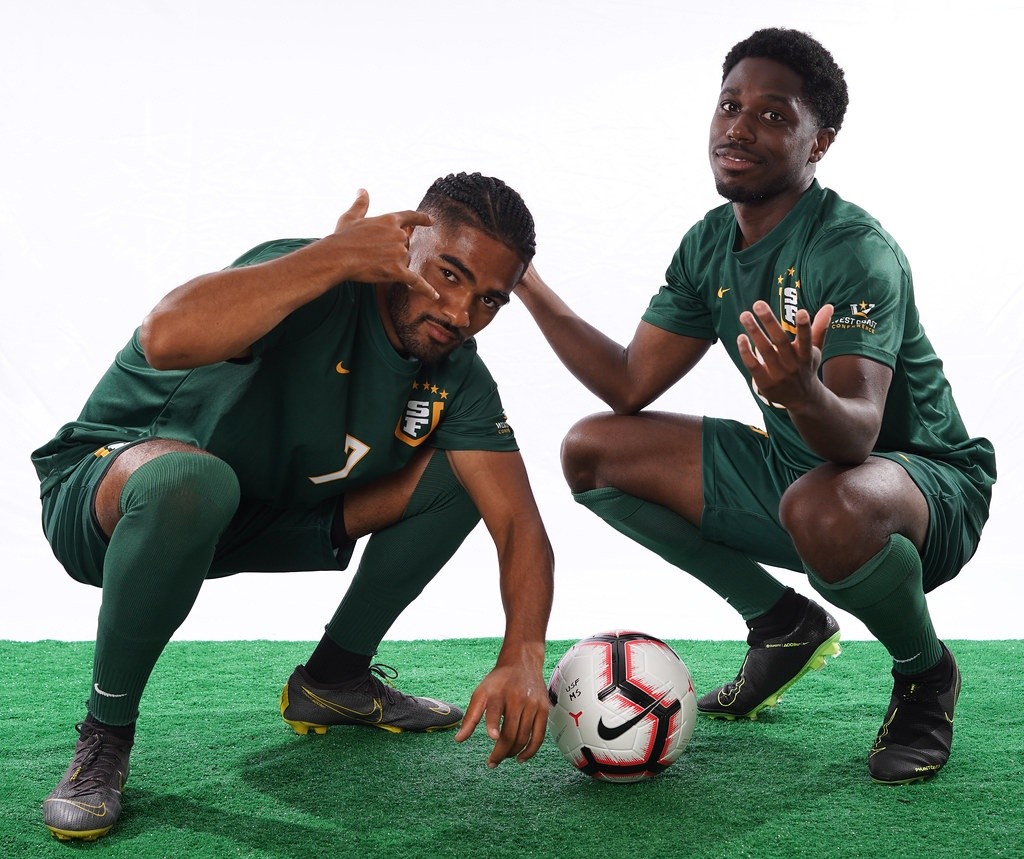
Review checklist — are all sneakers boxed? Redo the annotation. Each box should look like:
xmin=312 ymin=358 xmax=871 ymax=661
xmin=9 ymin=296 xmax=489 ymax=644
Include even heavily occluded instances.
xmin=42 ymin=731 xmax=132 ymax=843
xmin=279 ymin=664 xmax=463 ymax=735
xmin=867 ymin=638 xmax=963 ymax=785
xmin=695 ymin=597 xmax=842 ymax=721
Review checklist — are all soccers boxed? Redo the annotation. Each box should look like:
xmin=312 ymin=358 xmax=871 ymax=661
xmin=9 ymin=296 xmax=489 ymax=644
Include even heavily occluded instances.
xmin=545 ymin=632 xmax=699 ymax=787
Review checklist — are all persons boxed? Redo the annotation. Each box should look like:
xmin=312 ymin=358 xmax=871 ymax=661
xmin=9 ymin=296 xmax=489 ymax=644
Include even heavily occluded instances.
xmin=27 ymin=169 xmax=560 ymax=841
xmin=509 ymin=24 xmax=999 ymax=783
